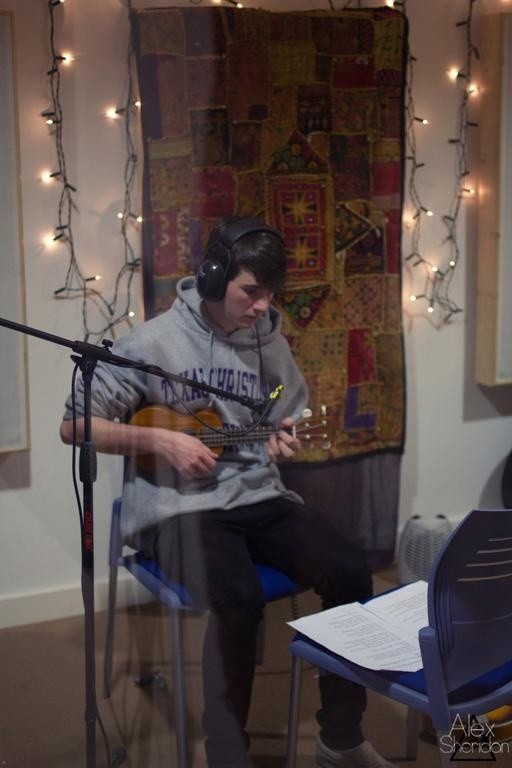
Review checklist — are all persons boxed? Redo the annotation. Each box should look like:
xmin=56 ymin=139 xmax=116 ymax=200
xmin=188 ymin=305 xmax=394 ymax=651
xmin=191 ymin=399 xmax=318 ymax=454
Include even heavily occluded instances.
xmin=58 ymin=216 xmax=403 ymax=768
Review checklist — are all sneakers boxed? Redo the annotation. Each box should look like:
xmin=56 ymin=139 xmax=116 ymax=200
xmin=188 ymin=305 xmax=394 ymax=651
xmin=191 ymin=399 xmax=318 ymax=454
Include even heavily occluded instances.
xmin=316 ymin=734 xmax=398 ymax=768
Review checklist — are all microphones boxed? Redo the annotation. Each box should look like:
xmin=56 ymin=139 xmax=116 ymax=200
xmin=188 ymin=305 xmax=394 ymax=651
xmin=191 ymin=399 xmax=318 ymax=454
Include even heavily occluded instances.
xmin=250 ymin=384 xmax=284 ymax=426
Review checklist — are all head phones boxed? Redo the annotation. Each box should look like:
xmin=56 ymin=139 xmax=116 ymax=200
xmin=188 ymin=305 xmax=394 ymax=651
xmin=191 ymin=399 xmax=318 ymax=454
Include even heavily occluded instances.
xmin=197 ymin=218 xmax=285 ymax=303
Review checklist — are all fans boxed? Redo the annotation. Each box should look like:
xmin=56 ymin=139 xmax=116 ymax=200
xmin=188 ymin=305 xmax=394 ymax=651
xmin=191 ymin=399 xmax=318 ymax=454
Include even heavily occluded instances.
xmin=397 ymin=514 xmax=452 ymax=588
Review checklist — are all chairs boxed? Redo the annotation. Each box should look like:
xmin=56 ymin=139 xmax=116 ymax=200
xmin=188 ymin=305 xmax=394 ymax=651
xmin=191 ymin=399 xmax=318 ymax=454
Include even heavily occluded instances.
xmin=102 ymin=497 xmax=316 ymax=766
xmin=284 ymin=509 xmax=511 ymax=767
